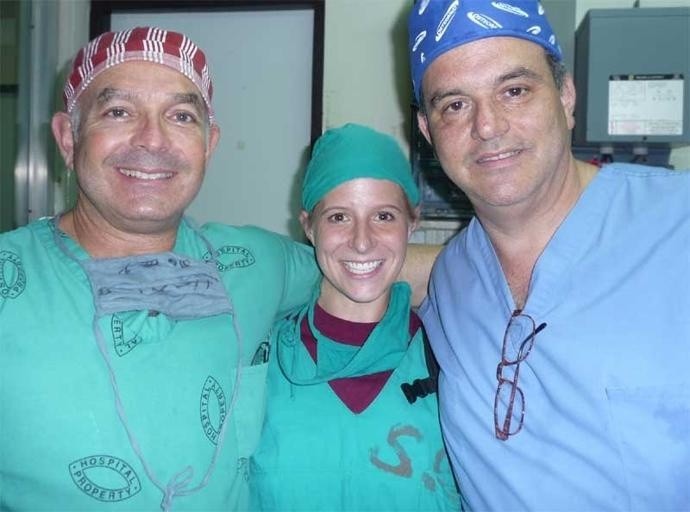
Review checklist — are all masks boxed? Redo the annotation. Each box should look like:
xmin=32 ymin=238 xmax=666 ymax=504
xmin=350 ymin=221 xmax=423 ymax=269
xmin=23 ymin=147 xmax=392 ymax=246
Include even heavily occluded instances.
xmin=277 ymin=281 xmax=412 ymax=385
xmin=80 ymin=251 xmax=233 ymax=319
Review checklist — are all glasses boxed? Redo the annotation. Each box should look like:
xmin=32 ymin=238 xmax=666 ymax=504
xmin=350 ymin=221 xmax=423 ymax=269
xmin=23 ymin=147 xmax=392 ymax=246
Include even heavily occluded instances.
xmin=494 ymin=311 xmax=547 ymax=440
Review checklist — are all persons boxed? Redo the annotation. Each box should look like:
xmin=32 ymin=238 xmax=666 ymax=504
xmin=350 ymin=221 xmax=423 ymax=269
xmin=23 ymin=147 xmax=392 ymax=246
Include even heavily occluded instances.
xmin=409 ymin=0 xmax=690 ymax=512
xmin=249 ymin=123 xmax=462 ymax=512
xmin=0 ymin=30 xmax=323 ymax=512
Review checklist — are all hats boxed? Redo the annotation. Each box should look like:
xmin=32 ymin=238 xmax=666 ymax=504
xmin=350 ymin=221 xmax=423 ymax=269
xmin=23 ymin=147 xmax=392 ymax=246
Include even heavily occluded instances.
xmin=62 ymin=26 xmax=214 ymax=124
xmin=409 ymin=0 xmax=566 ymax=109
xmin=302 ymin=123 xmax=420 ymax=216
